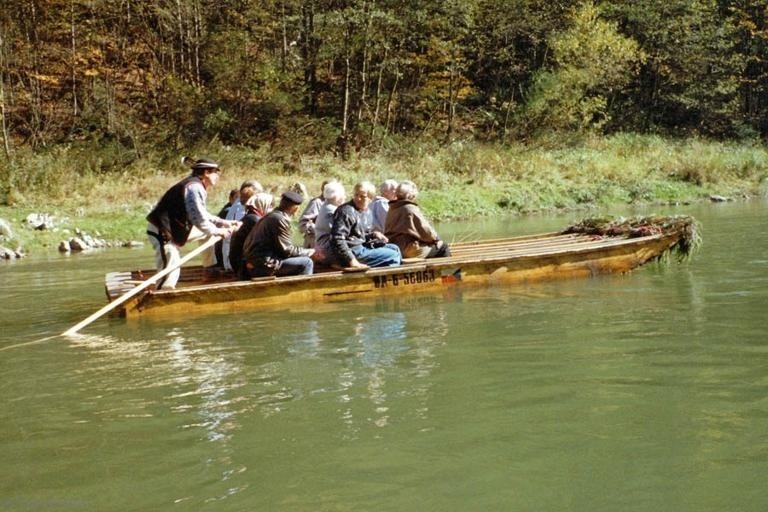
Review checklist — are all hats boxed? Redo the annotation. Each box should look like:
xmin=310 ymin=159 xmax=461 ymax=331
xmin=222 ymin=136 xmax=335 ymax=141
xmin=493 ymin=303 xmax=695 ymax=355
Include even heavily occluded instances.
xmin=190 ymin=158 xmax=221 ymax=171
xmin=282 ymin=192 xmax=303 ymax=205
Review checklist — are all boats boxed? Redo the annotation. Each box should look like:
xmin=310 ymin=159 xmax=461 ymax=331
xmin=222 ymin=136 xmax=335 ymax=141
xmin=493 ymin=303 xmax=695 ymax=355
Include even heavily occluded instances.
xmin=105 ymin=215 xmax=702 ymax=325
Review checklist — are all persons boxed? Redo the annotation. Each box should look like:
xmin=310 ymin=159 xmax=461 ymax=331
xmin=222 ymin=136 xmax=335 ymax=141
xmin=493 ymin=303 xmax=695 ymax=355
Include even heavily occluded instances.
xmin=222 ymin=179 xmax=263 ymax=270
xmin=215 ymin=189 xmax=239 ymax=267
xmin=228 ymin=193 xmax=276 ymax=276
xmin=144 ymin=159 xmax=236 ymax=291
xmin=235 ymin=190 xmax=255 ymax=221
xmin=300 ymin=181 xmax=328 ymax=249
xmin=384 ymin=180 xmax=452 ymax=258
xmin=312 ymin=180 xmax=349 ymax=266
xmin=242 ymin=192 xmax=317 ymax=276
xmin=369 ymin=180 xmax=399 ymax=230
xmin=329 ymin=182 xmax=402 ymax=270
